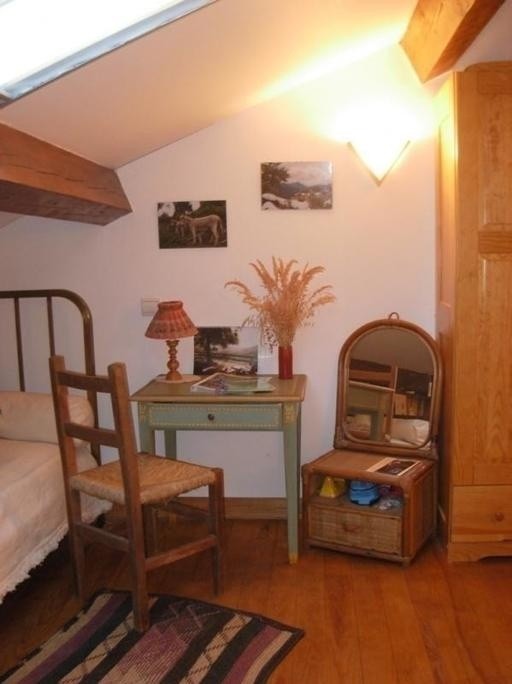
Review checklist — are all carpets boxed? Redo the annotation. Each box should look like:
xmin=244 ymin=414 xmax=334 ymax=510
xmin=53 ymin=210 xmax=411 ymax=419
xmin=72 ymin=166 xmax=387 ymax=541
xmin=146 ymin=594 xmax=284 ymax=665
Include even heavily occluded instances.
xmin=0 ymin=588 xmax=304 ymax=684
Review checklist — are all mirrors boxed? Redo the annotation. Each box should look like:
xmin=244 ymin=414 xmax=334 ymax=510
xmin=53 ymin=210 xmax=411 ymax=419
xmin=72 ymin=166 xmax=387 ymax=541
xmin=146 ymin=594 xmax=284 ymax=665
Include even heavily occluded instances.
xmin=332 ymin=310 xmax=443 ymax=461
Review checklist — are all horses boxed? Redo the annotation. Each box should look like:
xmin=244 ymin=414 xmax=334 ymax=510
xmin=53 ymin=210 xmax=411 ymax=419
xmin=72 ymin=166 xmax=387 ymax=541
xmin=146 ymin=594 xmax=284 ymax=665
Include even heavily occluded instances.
xmin=179 ymin=214 xmax=223 ymax=246
xmin=170 ymin=219 xmax=188 ymax=240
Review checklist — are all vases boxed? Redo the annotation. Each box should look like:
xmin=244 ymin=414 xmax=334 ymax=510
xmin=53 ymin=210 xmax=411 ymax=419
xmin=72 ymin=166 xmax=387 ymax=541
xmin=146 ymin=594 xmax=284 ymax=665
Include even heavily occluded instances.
xmin=278 ymin=346 xmax=294 ymax=379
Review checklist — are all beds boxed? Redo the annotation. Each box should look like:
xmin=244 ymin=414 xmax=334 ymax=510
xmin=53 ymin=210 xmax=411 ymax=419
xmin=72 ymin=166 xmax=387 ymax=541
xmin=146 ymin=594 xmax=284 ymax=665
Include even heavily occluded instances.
xmin=0 ymin=288 xmax=108 ymax=612
xmin=350 ymin=361 xmax=432 ymax=450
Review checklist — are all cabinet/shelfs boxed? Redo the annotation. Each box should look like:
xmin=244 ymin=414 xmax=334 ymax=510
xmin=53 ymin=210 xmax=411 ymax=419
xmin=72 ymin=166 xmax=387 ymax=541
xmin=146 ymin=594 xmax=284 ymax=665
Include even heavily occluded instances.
xmin=435 ymin=60 xmax=511 ymax=570
xmin=298 ymin=449 xmax=439 ymax=568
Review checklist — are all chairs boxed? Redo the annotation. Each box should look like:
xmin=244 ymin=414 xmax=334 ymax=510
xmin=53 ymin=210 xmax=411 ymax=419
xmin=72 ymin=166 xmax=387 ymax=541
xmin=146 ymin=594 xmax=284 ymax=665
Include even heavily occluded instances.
xmin=48 ymin=354 xmax=228 ymax=635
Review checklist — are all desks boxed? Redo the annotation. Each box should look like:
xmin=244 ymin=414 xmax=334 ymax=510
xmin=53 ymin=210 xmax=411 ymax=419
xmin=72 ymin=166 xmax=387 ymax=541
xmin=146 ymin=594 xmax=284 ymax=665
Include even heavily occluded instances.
xmin=131 ymin=373 xmax=309 ymax=567
xmin=343 ymin=376 xmax=396 ymax=445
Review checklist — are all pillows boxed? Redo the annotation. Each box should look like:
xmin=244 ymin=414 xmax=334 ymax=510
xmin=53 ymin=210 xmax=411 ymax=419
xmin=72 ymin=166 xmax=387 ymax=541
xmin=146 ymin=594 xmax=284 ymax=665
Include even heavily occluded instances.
xmin=0 ymin=388 xmax=97 ymax=449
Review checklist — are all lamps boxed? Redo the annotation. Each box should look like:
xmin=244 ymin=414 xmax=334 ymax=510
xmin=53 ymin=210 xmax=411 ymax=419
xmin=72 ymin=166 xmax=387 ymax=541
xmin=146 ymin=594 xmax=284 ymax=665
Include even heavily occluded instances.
xmin=145 ymin=302 xmax=201 ymax=382
